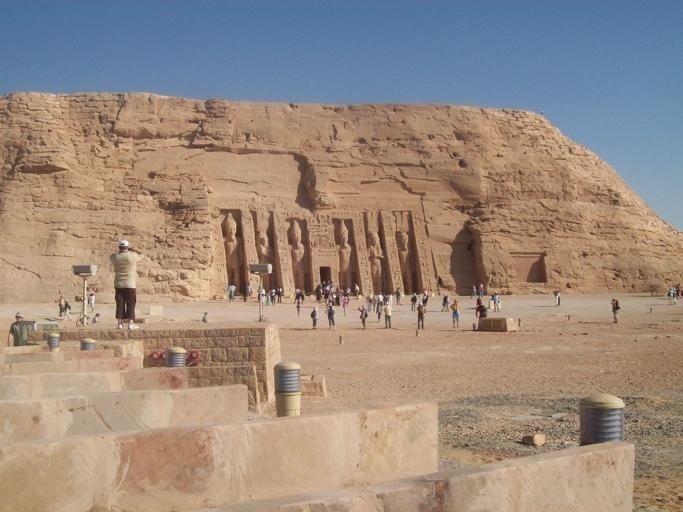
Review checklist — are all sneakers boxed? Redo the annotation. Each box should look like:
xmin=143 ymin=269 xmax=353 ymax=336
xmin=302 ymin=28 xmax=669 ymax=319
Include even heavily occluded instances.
xmin=127 ymin=323 xmax=139 ymax=329
xmin=117 ymin=323 xmax=125 ymax=329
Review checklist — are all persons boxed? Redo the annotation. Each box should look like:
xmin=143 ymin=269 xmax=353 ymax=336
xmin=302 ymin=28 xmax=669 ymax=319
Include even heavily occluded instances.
xmin=55 ymin=290 xmax=65 ymax=316
xmin=611 ymin=298 xmax=618 ymax=323
xmin=109 ymin=240 xmax=145 ymax=329
xmin=228 ymin=278 xmax=500 ymax=329
xmin=87 ymin=287 xmax=100 ymax=324
xmin=555 ymin=289 xmax=560 ymax=306
xmin=7 ymin=312 xmax=36 ymax=346
xmin=667 ymin=283 xmax=682 ymax=305
xmin=63 ymin=299 xmax=72 ymax=320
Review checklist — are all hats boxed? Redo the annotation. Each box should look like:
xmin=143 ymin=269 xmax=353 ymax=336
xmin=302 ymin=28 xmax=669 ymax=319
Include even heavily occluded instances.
xmin=118 ymin=240 xmax=129 ymax=247
